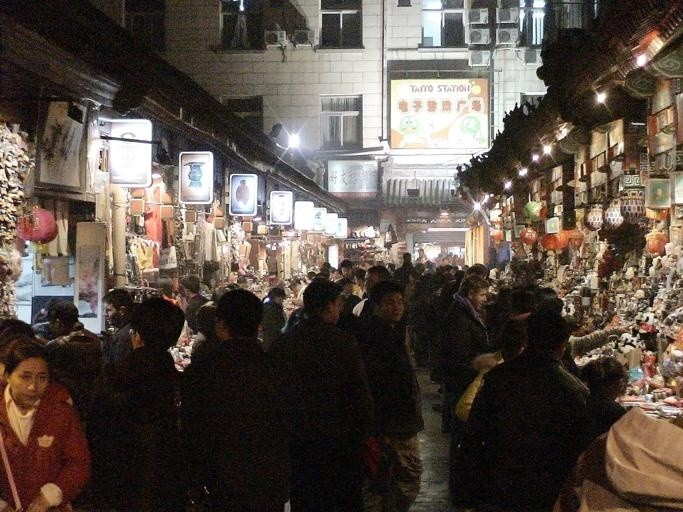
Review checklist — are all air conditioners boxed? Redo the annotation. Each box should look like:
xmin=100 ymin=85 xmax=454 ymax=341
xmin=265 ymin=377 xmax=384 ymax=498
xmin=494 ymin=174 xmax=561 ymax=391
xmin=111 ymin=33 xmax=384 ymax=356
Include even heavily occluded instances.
xmin=464 ymin=6 xmax=541 ymax=67
xmin=293 ymin=28 xmax=318 ymax=48
xmin=263 ymin=30 xmax=287 ymax=48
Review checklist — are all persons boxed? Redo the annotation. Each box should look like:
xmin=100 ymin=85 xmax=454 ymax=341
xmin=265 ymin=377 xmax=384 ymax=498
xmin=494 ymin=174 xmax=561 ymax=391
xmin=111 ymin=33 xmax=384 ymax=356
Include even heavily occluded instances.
xmin=1 ymin=243 xmax=682 ymax=511
